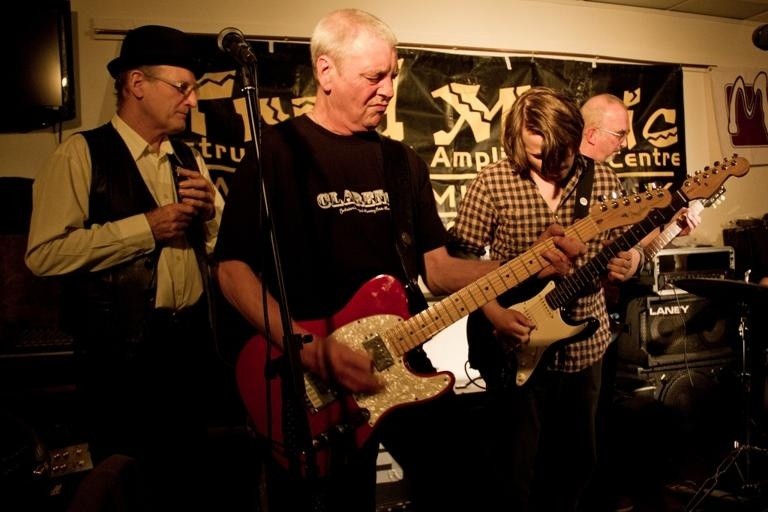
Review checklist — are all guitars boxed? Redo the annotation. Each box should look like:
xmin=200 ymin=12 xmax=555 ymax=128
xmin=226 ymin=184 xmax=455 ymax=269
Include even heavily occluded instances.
xmin=494 ymin=154 xmax=750 ymax=387
xmin=235 ymin=185 xmax=671 ymax=484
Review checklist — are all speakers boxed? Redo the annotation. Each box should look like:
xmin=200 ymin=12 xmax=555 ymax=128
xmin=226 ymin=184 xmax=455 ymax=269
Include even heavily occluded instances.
xmin=610 ymin=359 xmax=749 ymax=484
xmin=617 ymin=290 xmax=749 ymax=368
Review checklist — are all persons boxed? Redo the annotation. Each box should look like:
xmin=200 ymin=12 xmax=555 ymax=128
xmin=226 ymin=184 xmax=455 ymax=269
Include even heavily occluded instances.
xmin=25 ymin=26 xmax=226 ymax=512
xmin=449 ymin=88 xmax=645 ymax=512
xmin=577 ymin=93 xmax=700 ymax=512
xmin=212 ymin=10 xmax=590 ymax=511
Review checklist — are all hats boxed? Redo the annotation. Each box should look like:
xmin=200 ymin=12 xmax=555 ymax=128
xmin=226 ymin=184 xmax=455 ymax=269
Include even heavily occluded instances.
xmin=106 ymin=26 xmax=207 ymax=81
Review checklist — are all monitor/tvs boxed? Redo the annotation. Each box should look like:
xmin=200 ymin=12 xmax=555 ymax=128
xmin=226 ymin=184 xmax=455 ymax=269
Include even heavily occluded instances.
xmin=0 ymin=0 xmax=76 ymax=128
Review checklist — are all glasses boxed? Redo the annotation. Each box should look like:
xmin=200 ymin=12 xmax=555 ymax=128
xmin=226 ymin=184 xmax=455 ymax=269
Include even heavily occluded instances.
xmin=139 ymin=70 xmax=203 ymax=95
xmin=595 ymin=126 xmax=630 ymax=140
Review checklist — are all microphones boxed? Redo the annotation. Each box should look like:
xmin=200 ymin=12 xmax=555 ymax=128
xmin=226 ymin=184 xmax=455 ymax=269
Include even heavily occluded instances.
xmin=217 ymin=27 xmax=255 ymax=64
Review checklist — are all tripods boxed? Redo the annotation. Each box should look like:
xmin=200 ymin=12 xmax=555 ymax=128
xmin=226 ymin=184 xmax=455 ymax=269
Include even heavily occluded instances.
xmin=683 ymin=313 xmax=768 ymax=512
